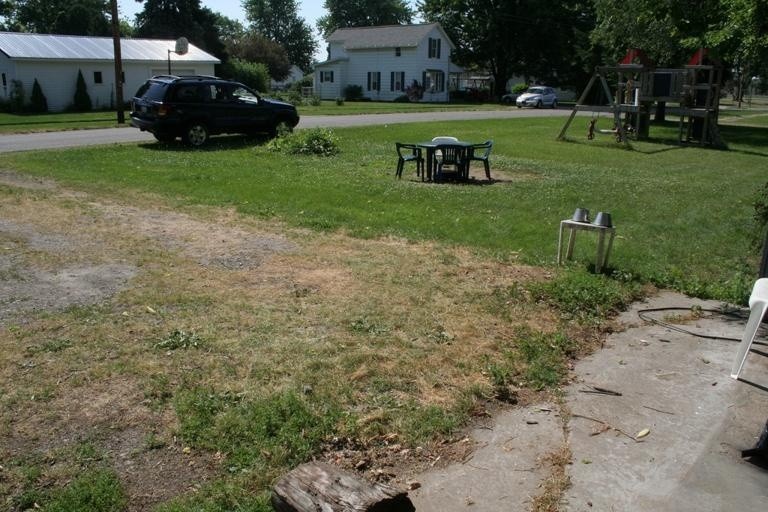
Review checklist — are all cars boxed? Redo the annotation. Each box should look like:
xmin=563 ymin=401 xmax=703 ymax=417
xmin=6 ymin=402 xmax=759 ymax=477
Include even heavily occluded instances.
xmin=502 ymin=86 xmax=558 ymax=108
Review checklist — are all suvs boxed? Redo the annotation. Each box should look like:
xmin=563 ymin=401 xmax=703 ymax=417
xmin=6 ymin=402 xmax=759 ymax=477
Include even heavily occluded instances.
xmin=129 ymin=74 xmax=300 ymax=147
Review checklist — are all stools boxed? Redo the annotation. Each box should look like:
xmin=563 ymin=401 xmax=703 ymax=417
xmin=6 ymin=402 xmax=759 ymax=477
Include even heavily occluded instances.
xmin=556 ymin=218 xmax=618 ymax=274
xmin=730 ymin=275 xmax=768 ymax=382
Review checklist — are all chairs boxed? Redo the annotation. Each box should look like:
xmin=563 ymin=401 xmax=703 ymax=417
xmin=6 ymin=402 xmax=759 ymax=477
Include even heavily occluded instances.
xmin=395 ymin=135 xmax=494 ymax=185
xmin=215 ymin=92 xmax=226 ymax=101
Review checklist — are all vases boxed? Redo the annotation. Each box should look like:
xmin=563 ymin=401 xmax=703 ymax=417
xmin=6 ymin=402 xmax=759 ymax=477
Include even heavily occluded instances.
xmin=572 ymin=207 xmax=613 ymax=228
